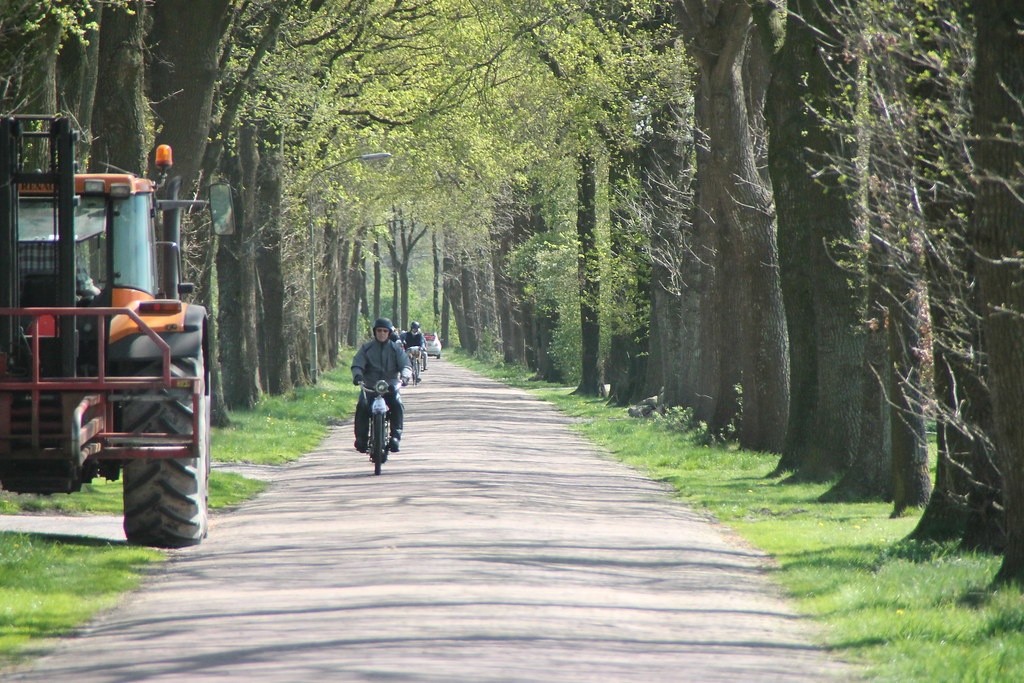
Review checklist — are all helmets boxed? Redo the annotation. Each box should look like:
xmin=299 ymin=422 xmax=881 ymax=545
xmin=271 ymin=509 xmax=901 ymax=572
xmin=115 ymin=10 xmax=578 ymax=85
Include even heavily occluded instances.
xmin=373 ymin=316 xmax=393 ymax=334
xmin=411 ymin=322 xmax=419 ymax=330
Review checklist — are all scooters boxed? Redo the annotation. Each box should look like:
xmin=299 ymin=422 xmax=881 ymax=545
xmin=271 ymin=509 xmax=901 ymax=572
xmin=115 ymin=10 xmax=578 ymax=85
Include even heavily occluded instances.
xmin=401 ymin=343 xmax=424 ymax=386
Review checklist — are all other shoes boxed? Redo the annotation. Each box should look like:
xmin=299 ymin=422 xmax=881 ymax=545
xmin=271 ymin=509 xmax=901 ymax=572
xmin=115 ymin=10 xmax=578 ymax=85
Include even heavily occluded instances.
xmin=354 ymin=440 xmax=367 ymax=454
xmin=389 ymin=436 xmax=399 ymax=452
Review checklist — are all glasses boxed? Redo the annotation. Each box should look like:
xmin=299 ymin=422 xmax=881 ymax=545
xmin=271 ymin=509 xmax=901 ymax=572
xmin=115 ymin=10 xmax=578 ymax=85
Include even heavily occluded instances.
xmin=375 ymin=328 xmax=389 ymax=333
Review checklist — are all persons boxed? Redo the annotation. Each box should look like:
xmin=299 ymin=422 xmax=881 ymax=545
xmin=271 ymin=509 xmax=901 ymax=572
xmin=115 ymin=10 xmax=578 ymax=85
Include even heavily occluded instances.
xmin=403 ymin=322 xmax=424 ymax=350
xmin=390 ymin=325 xmax=428 ymax=385
xmin=18 ymin=242 xmax=101 ymax=307
xmin=351 ymin=318 xmax=413 ymax=452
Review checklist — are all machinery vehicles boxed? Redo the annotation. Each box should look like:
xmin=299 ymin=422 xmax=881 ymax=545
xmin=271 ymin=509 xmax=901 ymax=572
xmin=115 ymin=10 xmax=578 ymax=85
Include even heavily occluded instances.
xmin=0 ymin=111 xmax=216 ymax=549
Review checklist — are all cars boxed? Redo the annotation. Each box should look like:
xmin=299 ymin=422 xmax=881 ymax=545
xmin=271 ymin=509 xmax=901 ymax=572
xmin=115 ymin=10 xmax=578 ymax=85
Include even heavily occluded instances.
xmin=424 ymin=332 xmax=443 ymax=359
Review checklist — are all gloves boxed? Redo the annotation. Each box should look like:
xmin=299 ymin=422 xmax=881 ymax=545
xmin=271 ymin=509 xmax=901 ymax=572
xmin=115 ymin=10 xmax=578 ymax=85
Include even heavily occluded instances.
xmin=353 ymin=374 xmax=365 ymax=386
xmin=400 ymin=377 xmax=408 ymax=387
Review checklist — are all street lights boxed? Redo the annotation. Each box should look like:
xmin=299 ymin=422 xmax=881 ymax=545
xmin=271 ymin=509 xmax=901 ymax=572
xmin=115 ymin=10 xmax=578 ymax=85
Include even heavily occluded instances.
xmin=306 ymin=150 xmax=391 ymax=385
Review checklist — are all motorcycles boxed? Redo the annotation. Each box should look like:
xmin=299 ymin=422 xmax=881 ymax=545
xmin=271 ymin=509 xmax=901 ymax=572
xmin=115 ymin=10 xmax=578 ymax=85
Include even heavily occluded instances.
xmin=357 ymin=378 xmax=404 ymax=475
